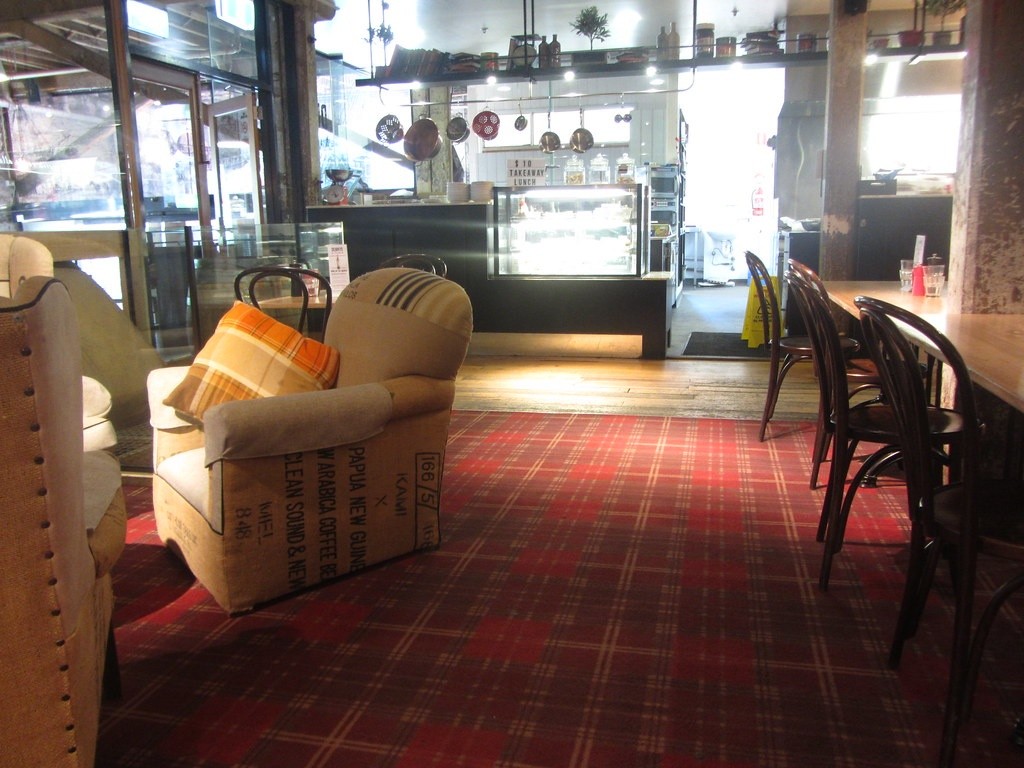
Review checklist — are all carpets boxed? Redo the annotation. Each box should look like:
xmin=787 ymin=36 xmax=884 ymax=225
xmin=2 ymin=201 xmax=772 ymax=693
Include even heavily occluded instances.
xmin=90 ymin=410 xmax=1024 ymax=768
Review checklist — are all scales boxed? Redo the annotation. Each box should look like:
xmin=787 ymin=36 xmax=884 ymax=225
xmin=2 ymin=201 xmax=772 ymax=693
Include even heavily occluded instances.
xmin=320 ymin=170 xmax=353 ymax=205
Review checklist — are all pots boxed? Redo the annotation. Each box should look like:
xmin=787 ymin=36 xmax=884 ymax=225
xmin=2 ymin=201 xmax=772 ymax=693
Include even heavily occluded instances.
xmin=472 ymin=111 xmax=499 ymax=139
xmin=446 ymin=117 xmax=470 ymax=143
xmin=514 ymin=116 xmax=528 ymax=131
xmin=570 ymin=128 xmax=594 ymax=154
xmin=403 ymin=118 xmax=443 ymax=161
xmin=539 ymin=132 xmax=561 ymax=154
xmin=375 ymin=115 xmax=404 ymax=143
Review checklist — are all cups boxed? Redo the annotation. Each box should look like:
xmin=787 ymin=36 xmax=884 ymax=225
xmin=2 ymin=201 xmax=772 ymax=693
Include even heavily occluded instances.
xmin=899 ymin=260 xmax=913 ymax=290
xmin=922 ymin=265 xmax=945 ymax=298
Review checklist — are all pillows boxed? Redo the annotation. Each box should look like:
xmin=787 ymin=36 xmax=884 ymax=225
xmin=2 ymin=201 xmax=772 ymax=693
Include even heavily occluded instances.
xmin=162 ymin=301 xmax=339 ymax=422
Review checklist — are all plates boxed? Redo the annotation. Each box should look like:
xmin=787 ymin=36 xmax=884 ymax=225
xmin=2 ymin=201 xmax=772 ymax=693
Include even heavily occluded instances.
xmin=447 ymin=181 xmax=495 ymax=203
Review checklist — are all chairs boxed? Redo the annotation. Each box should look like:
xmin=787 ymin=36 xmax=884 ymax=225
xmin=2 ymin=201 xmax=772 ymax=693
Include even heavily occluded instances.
xmin=786 ymin=259 xmax=905 ymax=490
xmin=855 ymin=295 xmax=1024 ymax=768
xmin=744 ymin=250 xmax=860 ymax=442
xmin=234 ymin=266 xmax=332 ymax=344
xmin=377 ymin=253 xmax=447 ymax=278
xmin=783 ymin=270 xmax=985 ymax=593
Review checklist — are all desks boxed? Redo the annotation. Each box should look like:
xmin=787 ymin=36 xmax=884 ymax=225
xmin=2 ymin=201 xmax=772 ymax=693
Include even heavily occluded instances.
xmin=250 ymin=290 xmax=339 ymax=344
xmin=819 ymin=281 xmax=1024 ymax=561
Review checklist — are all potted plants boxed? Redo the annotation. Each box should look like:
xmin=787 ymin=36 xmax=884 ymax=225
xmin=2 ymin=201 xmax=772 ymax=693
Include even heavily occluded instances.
xmin=568 ymin=4 xmax=611 ymax=67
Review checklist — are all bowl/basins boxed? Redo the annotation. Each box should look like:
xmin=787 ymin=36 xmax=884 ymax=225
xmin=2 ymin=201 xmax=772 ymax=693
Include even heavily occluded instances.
xmin=799 ymin=220 xmax=820 ymax=231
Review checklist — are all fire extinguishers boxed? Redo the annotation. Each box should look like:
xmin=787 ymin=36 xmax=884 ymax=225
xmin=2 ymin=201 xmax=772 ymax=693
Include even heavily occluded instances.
xmin=751 ymin=186 xmax=764 ymax=217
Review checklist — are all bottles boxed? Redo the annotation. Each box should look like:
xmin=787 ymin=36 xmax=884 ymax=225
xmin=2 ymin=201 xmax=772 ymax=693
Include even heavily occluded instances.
xmin=656 ymin=22 xmax=680 ymax=60
xmin=538 ymin=34 xmax=561 ymax=68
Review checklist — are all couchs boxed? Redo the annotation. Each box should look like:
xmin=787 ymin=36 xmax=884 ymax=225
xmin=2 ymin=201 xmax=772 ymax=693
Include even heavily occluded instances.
xmin=0 ymin=276 xmax=122 ymax=768
xmin=145 ymin=267 xmax=473 ymax=614
xmin=0 ymin=233 xmax=112 ymax=422
